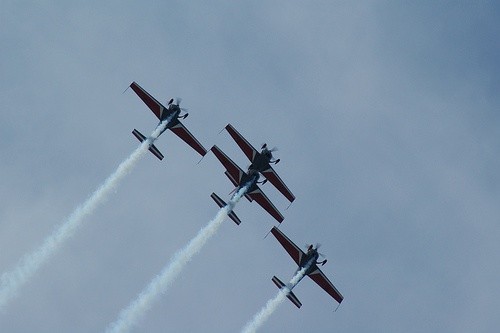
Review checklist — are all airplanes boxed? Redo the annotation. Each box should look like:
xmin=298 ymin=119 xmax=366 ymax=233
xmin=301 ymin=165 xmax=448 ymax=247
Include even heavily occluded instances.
xmin=130 ymin=82 xmax=207 ymax=160
xmin=210 ymin=144 xmax=285 ymax=224
xmin=270 ymin=226 xmax=343 ymax=308
xmin=225 ymin=123 xmax=295 ymax=202
